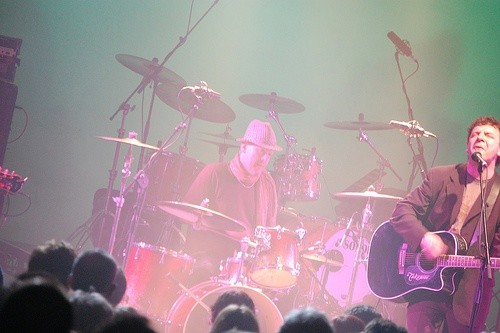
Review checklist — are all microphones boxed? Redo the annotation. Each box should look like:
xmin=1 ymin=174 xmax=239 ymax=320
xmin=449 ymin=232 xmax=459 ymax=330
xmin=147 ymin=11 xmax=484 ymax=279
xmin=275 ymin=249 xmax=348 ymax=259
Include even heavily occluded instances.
xmin=389 ymin=120 xmax=437 ymax=141
xmin=471 ymin=152 xmax=488 ymax=170
xmin=387 ymin=32 xmax=417 ymax=61
xmin=188 ymin=85 xmax=221 ymax=99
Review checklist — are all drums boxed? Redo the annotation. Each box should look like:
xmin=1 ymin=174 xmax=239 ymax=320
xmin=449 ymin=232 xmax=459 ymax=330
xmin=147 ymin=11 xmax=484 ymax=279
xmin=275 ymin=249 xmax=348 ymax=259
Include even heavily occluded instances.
xmin=122 ymin=240 xmax=198 ymax=322
xmin=273 ymin=154 xmax=321 ymax=201
xmin=249 ymin=226 xmax=301 ymax=289
xmin=151 ymin=152 xmax=207 ymax=205
xmin=165 ymin=278 xmax=285 ymax=332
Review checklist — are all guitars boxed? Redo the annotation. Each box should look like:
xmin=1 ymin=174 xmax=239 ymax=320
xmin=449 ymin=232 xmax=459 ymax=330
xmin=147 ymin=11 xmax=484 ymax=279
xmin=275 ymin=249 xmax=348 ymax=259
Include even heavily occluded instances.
xmin=367 ymin=217 xmax=500 ymax=304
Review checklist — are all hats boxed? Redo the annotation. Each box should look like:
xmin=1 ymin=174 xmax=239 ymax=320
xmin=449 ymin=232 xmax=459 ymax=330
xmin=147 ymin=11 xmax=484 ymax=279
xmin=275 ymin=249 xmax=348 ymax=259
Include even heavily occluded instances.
xmin=237 ymin=120 xmax=283 ymax=152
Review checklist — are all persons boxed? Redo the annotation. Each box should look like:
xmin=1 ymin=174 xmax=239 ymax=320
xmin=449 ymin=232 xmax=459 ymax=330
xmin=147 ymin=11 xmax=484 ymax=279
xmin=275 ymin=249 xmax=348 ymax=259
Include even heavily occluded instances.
xmin=177 ymin=118 xmax=277 ymax=280
xmin=0 ymin=237 xmax=410 ymax=333
xmin=389 ymin=115 xmax=500 ymax=333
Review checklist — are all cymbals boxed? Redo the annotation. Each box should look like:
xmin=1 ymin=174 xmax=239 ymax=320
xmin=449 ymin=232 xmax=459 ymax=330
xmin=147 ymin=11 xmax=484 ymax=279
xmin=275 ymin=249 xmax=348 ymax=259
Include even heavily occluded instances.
xmin=199 ymin=129 xmax=243 ymax=140
xmin=204 ymin=139 xmax=239 ymax=149
xmin=155 ymin=84 xmax=236 ymax=124
xmin=300 ymin=253 xmax=342 ymax=268
xmin=114 ymin=53 xmax=188 ymax=88
xmin=95 ymin=135 xmax=162 ymax=151
xmin=331 ymin=190 xmax=404 ymax=205
xmin=323 ymin=120 xmax=398 ymax=131
xmin=238 ymin=93 xmax=306 ymax=114
xmin=155 ymin=200 xmax=247 ymax=232
